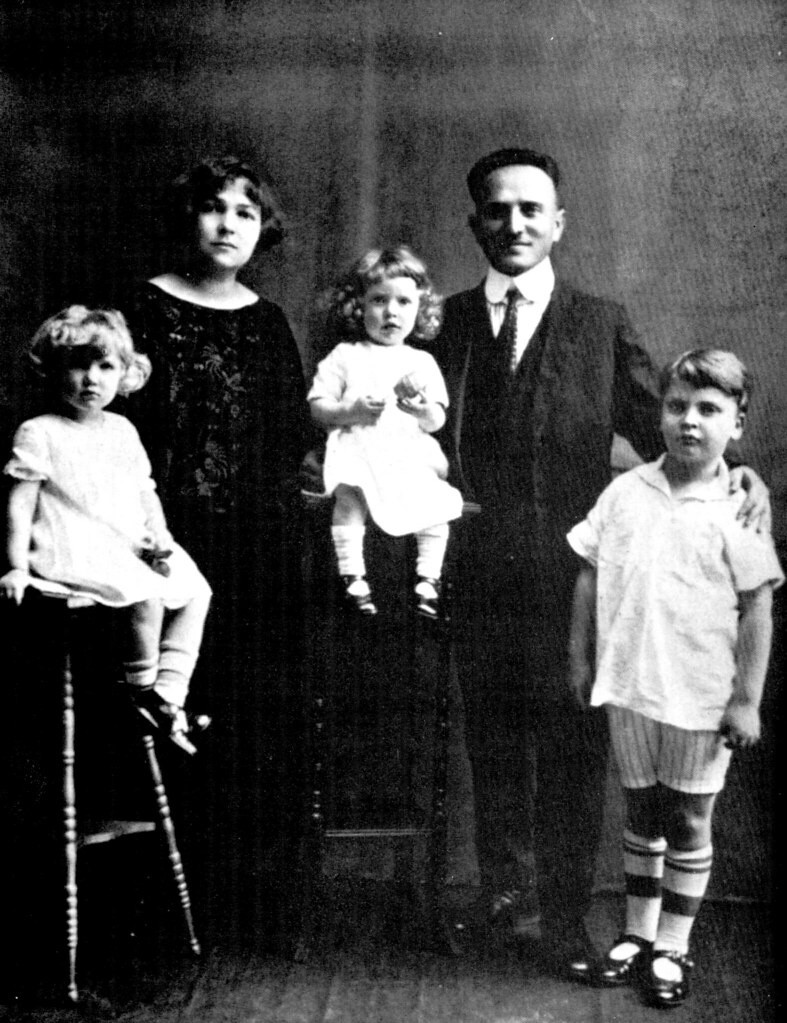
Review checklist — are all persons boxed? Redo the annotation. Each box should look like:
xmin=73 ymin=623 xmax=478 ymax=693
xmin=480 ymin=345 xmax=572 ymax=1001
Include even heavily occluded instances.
xmin=3 ymin=300 xmax=212 ymax=757
xmin=122 ymin=139 xmax=320 ymax=947
xmin=409 ymin=146 xmax=771 ymax=978
xmin=562 ymin=342 xmax=787 ymax=1008
xmin=299 ymin=243 xmax=464 ymax=624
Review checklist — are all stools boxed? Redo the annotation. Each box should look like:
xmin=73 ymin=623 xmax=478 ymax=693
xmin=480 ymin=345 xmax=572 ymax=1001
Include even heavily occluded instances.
xmin=0 ymin=582 xmax=200 ymax=1005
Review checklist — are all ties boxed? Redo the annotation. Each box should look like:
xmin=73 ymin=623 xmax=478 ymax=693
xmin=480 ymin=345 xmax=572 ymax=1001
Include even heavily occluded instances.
xmin=495 ymin=288 xmax=524 ymax=376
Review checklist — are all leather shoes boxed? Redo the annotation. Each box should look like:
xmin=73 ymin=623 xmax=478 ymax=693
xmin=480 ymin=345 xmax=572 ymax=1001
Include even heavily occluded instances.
xmin=596 ymin=933 xmax=651 ymax=985
xmin=453 ymin=875 xmax=541 ymax=934
xmin=643 ymin=942 xmax=695 ymax=1008
xmin=559 ymin=891 xmax=624 ymax=985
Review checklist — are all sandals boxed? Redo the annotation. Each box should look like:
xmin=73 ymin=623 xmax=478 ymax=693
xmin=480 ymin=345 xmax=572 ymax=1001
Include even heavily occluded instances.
xmin=410 ymin=576 xmax=442 ymax=620
xmin=136 ymin=688 xmax=197 ymax=757
xmin=339 ymin=574 xmax=379 ymax=617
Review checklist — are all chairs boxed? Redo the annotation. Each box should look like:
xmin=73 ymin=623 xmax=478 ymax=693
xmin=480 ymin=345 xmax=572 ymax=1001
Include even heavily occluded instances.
xmin=290 ymin=335 xmax=475 ymax=963
